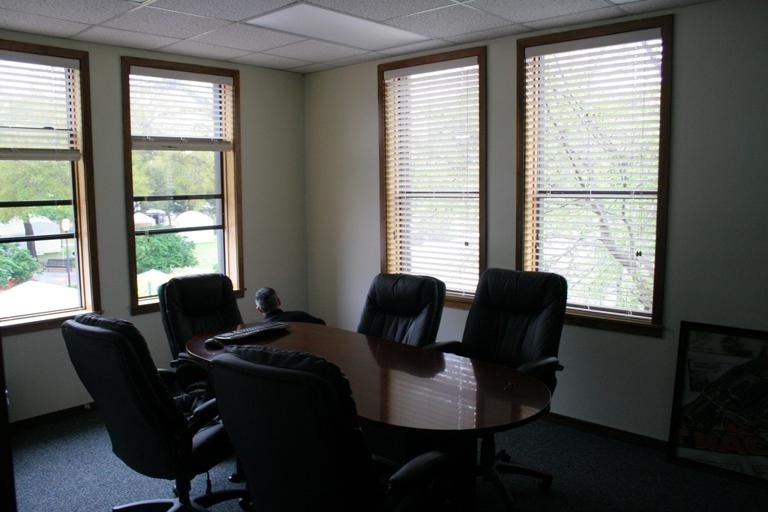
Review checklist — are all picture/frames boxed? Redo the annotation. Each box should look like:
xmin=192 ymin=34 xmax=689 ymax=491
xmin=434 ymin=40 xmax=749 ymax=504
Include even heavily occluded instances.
xmin=662 ymin=317 xmax=767 ymax=488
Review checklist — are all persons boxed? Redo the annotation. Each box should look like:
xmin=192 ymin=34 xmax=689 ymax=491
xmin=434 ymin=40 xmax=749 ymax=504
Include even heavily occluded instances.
xmin=252 ymin=286 xmax=327 ymax=327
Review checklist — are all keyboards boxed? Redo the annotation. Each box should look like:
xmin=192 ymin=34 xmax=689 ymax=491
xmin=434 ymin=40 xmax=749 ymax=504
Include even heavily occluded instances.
xmin=214 ymin=322 xmax=288 ymax=345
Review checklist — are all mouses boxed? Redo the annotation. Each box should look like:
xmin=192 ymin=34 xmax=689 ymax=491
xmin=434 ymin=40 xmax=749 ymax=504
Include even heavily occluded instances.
xmin=204 ymin=339 xmax=222 ymax=350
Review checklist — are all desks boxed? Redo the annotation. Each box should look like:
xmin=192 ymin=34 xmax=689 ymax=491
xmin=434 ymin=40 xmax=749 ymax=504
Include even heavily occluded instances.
xmin=183 ymin=317 xmax=556 ymax=511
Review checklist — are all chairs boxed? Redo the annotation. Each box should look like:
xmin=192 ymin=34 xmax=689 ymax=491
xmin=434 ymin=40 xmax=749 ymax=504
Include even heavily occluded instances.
xmin=61 ymin=310 xmax=247 ymax=512
xmin=207 ymin=343 xmax=450 ymax=512
xmin=351 ymin=270 xmax=448 ymax=348
xmin=418 ymin=265 xmax=570 ymax=507
xmin=157 ymin=272 xmax=248 ymax=389
